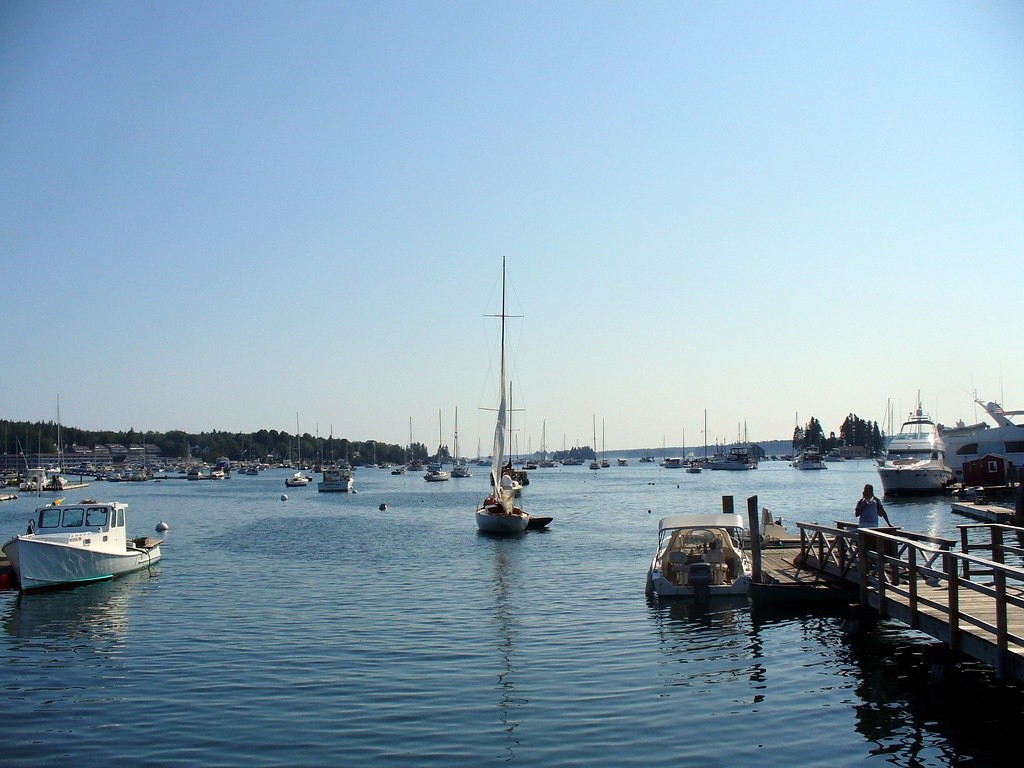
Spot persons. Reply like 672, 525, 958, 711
1006, 461, 1017, 486
703, 542, 726, 564
855, 484, 893, 569
26, 520, 35, 535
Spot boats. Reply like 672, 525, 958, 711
685, 460, 703, 472
2, 498, 163, 591
794, 444, 829, 471
317, 465, 354, 492
639, 455, 656, 463
618, 459, 627, 466
642, 513, 754, 600
526, 517, 554, 528
0, 407, 583, 489
658, 409, 760, 470
823, 447, 846, 462
876, 387, 953, 496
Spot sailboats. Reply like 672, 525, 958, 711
600, 417, 611, 467
472, 255, 531, 534
450, 405, 472, 478
285, 411, 309, 487
513, 418, 586, 470
588, 413, 601, 469
421, 408, 450, 481
